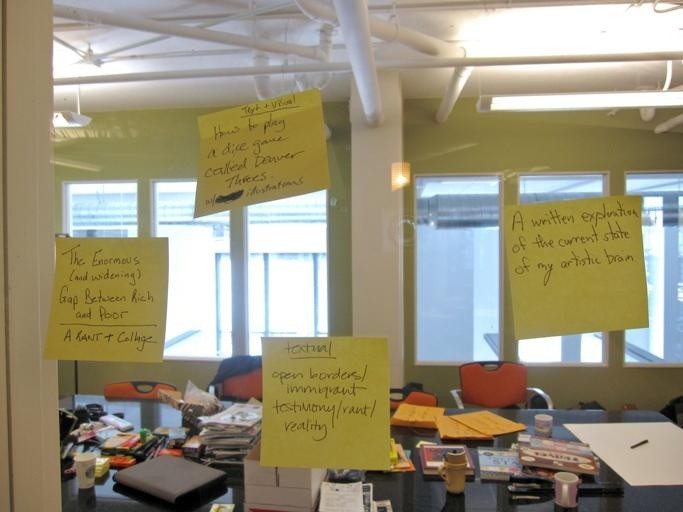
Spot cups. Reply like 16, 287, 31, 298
440, 454, 468, 494
534, 414, 554, 437
555, 471, 580, 508
73, 454, 96, 489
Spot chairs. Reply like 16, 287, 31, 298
451, 362, 554, 411
103, 381, 176, 399
209, 356, 263, 401
386, 389, 439, 408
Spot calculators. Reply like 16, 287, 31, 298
506, 475, 553, 504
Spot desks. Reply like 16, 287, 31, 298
58, 394, 683, 512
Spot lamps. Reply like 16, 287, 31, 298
476, 87, 683, 112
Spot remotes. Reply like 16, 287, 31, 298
99, 414, 133, 431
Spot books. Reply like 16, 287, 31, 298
113, 454, 228, 505
243, 442, 330, 512
197, 403, 263, 464
478, 432, 597, 485
419, 443, 475, 476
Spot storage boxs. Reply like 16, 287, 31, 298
243, 442, 330, 512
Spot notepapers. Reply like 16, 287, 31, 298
390, 403, 525, 441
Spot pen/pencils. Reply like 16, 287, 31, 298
630, 440, 648, 448
135, 436, 170, 463
579, 488, 624, 496
